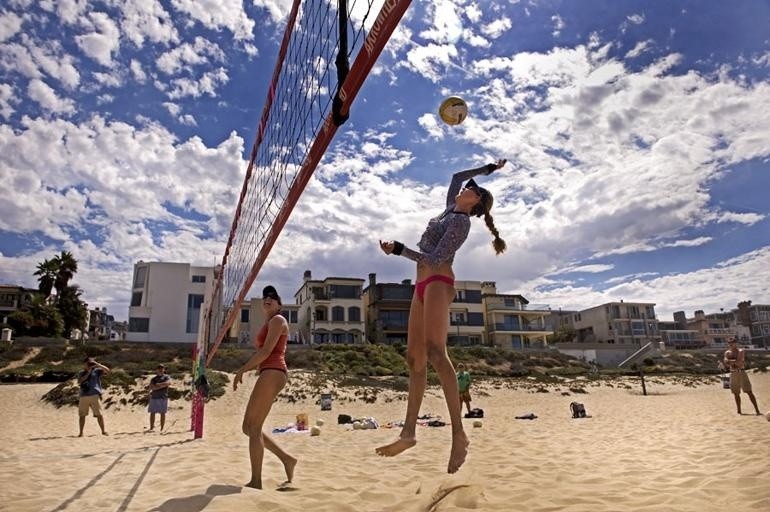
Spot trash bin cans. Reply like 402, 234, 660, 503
2, 328, 12, 341
322, 394, 331, 411
723, 377, 730, 389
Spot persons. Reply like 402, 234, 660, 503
76, 356, 111, 436
231, 285, 299, 491
722, 337, 761, 416
147, 362, 173, 432
374, 156, 509, 476
455, 362, 472, 417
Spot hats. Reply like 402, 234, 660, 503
262, 285, 281, 304
464, 178, 493, 218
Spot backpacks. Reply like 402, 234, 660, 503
465, 408, 484, 418
569, 402, 586, 419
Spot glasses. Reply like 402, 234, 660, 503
263, 293, 277, 300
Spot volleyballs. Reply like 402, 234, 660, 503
472, 419, 483, 428
311, 425, 322, 436
439, 95, 467, 125
316, 419, 324, 426
353, 422, 362, 430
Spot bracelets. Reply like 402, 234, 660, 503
485, 163, 497, 176
391, 240, 406, 256
